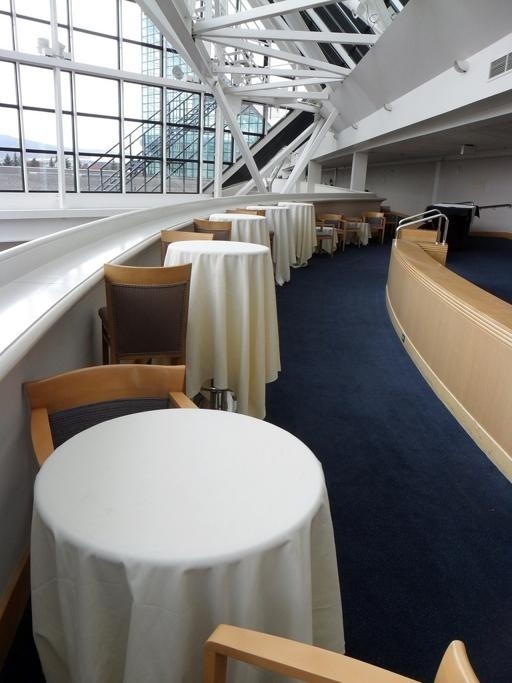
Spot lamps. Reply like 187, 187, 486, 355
384, 104, 392, 111
352, 121, 359, 129
454, 60, 469, 73
